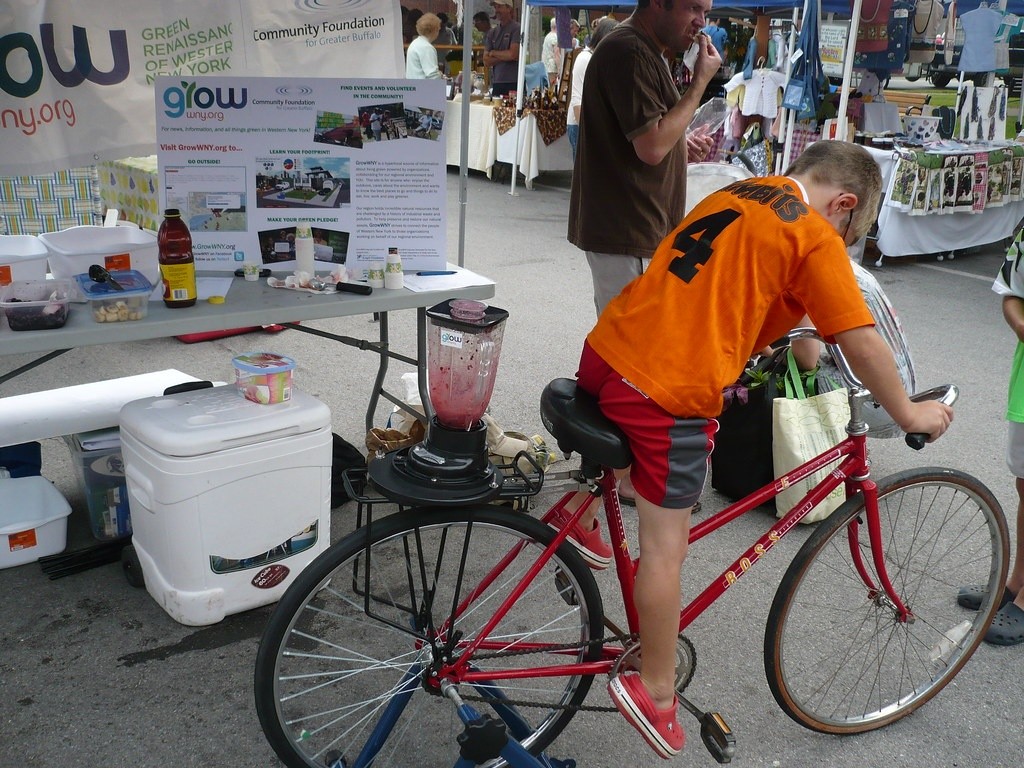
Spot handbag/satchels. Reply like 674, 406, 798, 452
782, 71, 831, 121
711, 346, 852, 524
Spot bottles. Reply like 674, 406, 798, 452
854, 132, 893, 149
455, 71, 464, 97
440, 74, 454, 100
504, 93, 517, 106
473, 74, 484, 93
551, 85, 560, 110
157, 209, 198, 307
534, 91, 542, 109
543, 91, 550, 110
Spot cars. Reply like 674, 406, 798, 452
922, 28, 1024, 98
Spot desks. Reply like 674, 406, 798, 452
441, 97, 575, 195
806, 135, 1024, 266
0, 264, 496, 454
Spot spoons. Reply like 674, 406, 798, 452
91, 265, 128, 290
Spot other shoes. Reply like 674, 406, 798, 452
617, 487, 702, 514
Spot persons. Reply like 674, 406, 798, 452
758, 258, 914, 438
700, 17, 729, 100
567, 0, 721, 324
401, 6, 458, 74
547, 140, 953, 759
957, 226, 1024, 646
413, 110, 432, 138
936, 35, 942, 42
567, 19, 621, 161
541, 17, 580, 87
482, 0, 520, 97
362, 107, 390, 141
405, 14, 443, 79
473, 12, 491, 66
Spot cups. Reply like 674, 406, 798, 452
482, 99, 502, 107
241, 261, 260, 281
368, 254, 404, 290
294, 222, 316, 278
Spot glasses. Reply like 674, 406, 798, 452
473, 21, 487, 24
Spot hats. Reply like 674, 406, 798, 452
489, 0, 514, 9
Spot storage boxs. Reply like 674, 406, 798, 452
118, 383, 333, 624
1, 225, 158, 322
0, 475, 72, 570
61, 433, 134, 541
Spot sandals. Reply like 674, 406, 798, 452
981, 600, 1024, 646
547, 507, 614, 570
956, 584, 1016, 612
606, 673, 686, 759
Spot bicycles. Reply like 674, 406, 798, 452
252, 320, 1014, 768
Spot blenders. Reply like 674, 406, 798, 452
365, 297, 504, 511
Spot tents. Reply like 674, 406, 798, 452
510, 0, 853, 193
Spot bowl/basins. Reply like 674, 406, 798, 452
900, 115, 943, 142
75, 269, 155, 322
0, 278, 74, 331
